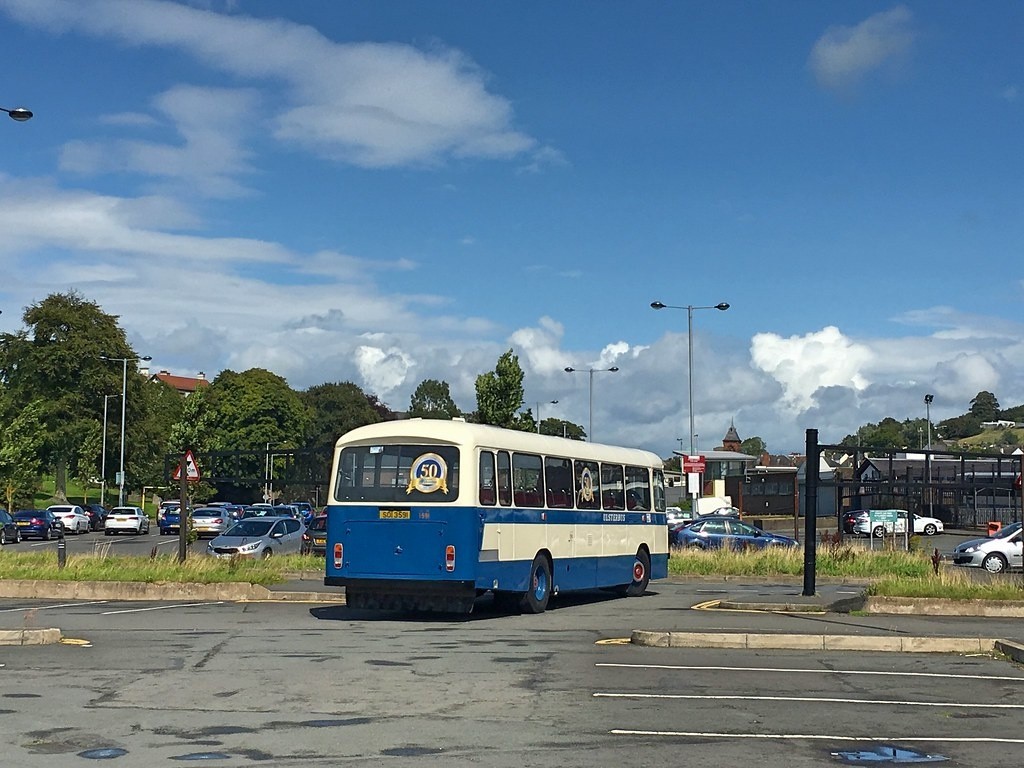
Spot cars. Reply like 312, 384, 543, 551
666, 507, 801, 551
842, 509, 945, 537
104, 506, 151, 536
0, 502, 103, 546
950, 521, 1024, 573
157, 500, 330, 565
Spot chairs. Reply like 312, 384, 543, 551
481, 485, 638, 510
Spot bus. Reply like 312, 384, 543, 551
324, 418, 671, 614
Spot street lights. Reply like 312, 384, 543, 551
99, 354, 152, 507
650, 300, 731, 456
564, 366, 619, 442
923, 394, 935, 517
676, 438, 684, 458
693, 434, 699, 451
264, 440, 296, 503
521, 401, 558, 434
